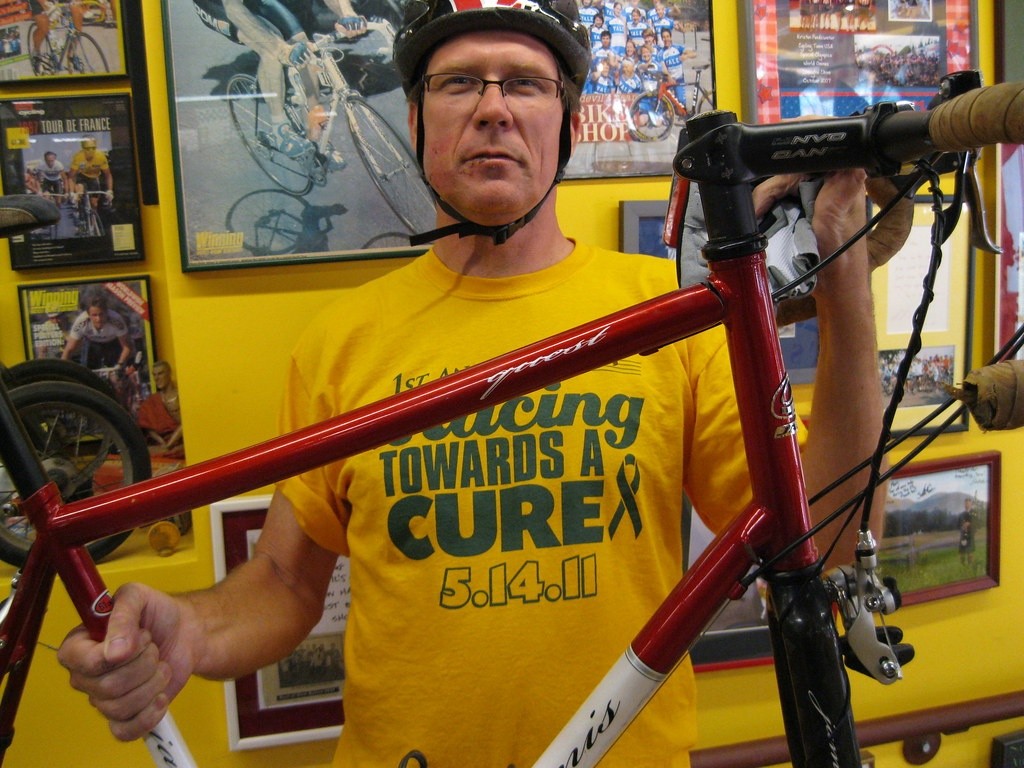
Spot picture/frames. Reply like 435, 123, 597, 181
881, 450, 1000, 608
618, 202, 872, 387
682, 501, 777, 672
210, 496, 352, 749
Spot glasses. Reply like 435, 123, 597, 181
83, 146, 97, 151
421, 72, 565, 114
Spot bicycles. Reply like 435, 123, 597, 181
71, 191, 113, 237
627, 64, 712, 143
0, 70, 1024, 768
227, 29, 438, 235
27, 2, 110, 77
38, 194, 69, 239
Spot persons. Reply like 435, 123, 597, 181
29, 0, 84, 76
800, 0, 938, 87
25, 136, 114, 237
878, 351, 951, 395
35, 294, 134, 410
56, 0, 891, 768
578, 0, 686, 125
192, 0, 367, 171
142, 360, 183, 450
956, 498, 980, 565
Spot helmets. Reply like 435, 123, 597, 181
391, 0, 592, 97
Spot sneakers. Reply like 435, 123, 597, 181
268, 119, 316, 159
318, 143, 346, 171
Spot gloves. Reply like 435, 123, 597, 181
70, 192, 78, 205
37, 191, 43, 197
105, 189, 114, 201
64, 192, 69, 198
334, 14, 368, 39
280, 40, 315, 72
